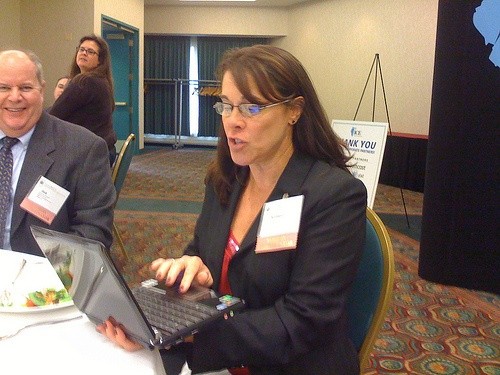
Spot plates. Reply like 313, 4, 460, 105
0, 269, 75, 313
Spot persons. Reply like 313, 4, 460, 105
96, 46, 368, 375
54, 77, 71, 99
52, 36, 117, 167
0, 50, 116, 258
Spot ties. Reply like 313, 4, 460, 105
0, 136, 20, 250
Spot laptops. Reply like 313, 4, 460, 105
29, 224, 247, 351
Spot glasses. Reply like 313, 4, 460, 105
76, 47, 99, 57
212, 98, 294, 119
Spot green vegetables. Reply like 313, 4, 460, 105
28, 289, 71, 307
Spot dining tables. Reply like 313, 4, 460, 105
0, 248, 167, 375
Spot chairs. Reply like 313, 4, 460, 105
110, 133, 136, 264
359, 206, 395, 375
